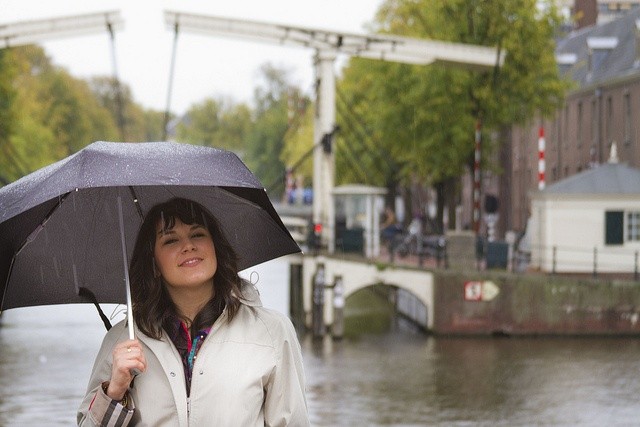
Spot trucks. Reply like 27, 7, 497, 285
281, 217, 307, 241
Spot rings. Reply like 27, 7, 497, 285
126, 345, 132, 352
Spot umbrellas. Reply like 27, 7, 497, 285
0, 138, 305, 375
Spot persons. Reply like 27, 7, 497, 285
77, 197, 313, 427
379, 205, 398, 264
